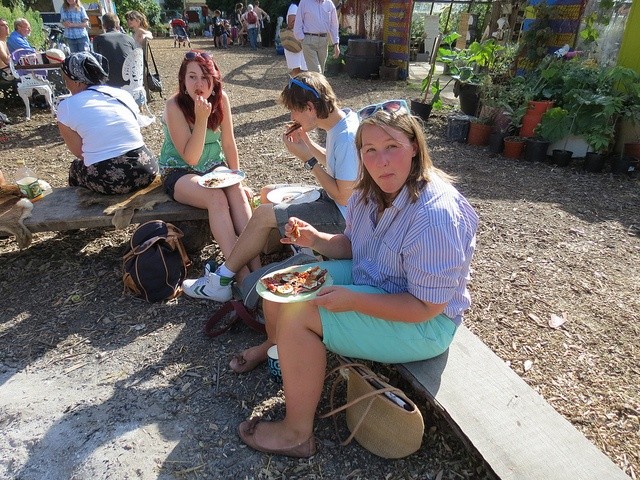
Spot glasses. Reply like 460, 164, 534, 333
357, 99, 409, 123
287, 73, 319, 98
126, 16, 136, 21
184, 52, 213, 62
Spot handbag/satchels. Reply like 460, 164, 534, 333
279, 26, 302, 53
145, 41, 163, 100
316, 355, 426, 458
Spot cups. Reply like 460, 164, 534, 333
25, 55, 37, 66
18, 57, 27, 66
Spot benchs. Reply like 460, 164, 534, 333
3, 188, 232, 255
269, 185, 633, 477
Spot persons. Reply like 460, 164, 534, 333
57, 52, 157, 197
234, 2, 246, 44
0, 23, 11, 71
121, 9, 151, 48
92, 9, 138, 87
281, 0, 303, 78
227, 102, 478, 460
160, 49, 262, 284
241, 3, 259, 49
57, 0, 92, 56
238, 21, 247, 35
293, 1, 342, 78
7, 18, 54, 82
251, 0, 268, 49
212, 9, 226, 49
182, 73, 366, 304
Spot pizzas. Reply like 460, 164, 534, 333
285, 219, 303, 242
285, 122, 302, 136
259, 264, 328, 298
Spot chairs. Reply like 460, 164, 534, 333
106, 48, 156, 110
47, 23, 65, 45
143, 37, 153, 104
9, 49, 57, 120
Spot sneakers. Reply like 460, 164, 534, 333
237, 416, 316, 458
181, 263, 232, 302
229, 345, 268, 373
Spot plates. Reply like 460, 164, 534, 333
255, 267, 334, 304
266, 187, 321, 206
198, 169, 246, 189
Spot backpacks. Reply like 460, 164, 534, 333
120, 218, 192, 302
204, 251, 319, 338
246, 11, 258, 24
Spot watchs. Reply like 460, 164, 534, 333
304, 157, 318, 171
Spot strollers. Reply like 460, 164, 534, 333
171, 19, 190, 48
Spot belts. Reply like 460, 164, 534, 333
305, 32, 328, 37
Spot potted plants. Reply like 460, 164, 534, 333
326, 45, 345, 74
410, 31, 497, 116
535, 84, 595, 167
585, 66, 626, 170
470, 79, 517, 145
501, 94, 531, 158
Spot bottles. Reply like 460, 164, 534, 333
15, 160, 43, 202
